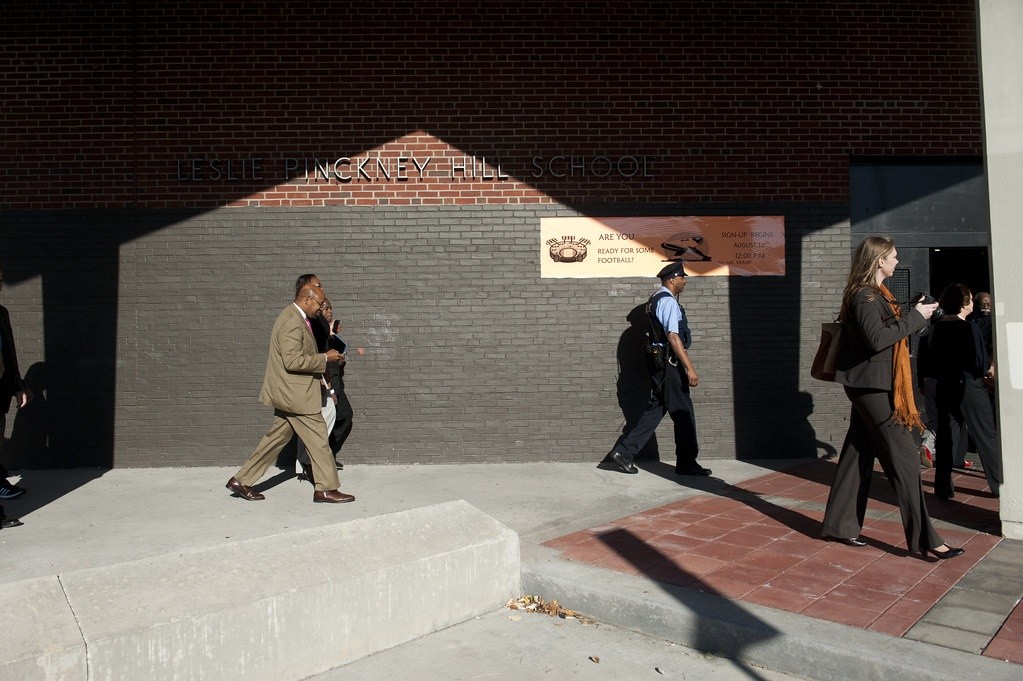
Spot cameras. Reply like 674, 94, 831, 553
912, 293, 945, 324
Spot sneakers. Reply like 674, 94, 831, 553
0, 478, 26, 499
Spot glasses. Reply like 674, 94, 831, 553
313, 298, 323, 307
315, 283, 322, 289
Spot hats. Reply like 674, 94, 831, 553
657, 261, 688, 282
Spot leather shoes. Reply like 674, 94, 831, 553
0, 517, 21, 528
676, 462, 712, 476
609, 450, 638, 474
226, 476, 265, 501
313, 489, 355, 503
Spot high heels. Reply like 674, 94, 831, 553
827, 536, 867, 546
922, 544, 964, 559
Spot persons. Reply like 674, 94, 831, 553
810, 235, 965, 561
916, 284, 1003, 510
0, 281, 29, 528
225, 273, 355, 504
596, 262, 712, 477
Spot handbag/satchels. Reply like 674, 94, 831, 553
811, 312, 843, 381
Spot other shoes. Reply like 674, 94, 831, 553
920, 446, 933, 468
302, 463, 314, 484
964, 460, 973, 468
335, 461, 343, 468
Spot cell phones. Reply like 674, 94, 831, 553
342, 352, 347, 355
